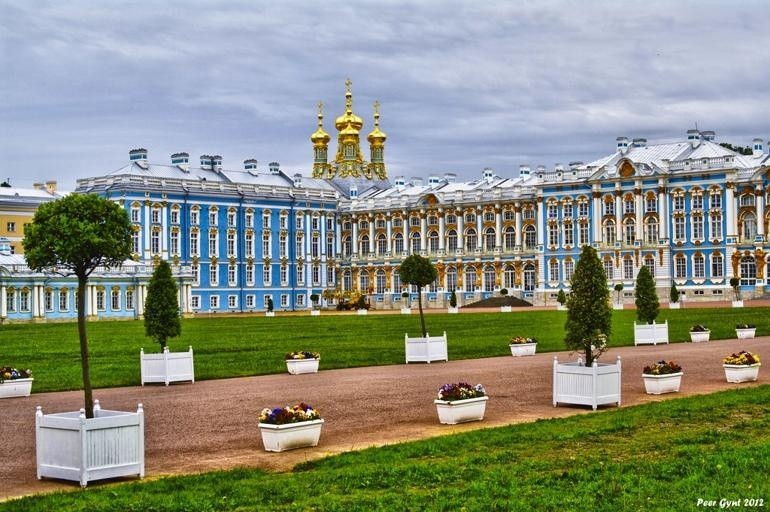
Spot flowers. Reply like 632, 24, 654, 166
436, 381, 486, 402
641, 360, 682, 376
736, 324, 755, 329
689, 325, 709, 332
258, 402, 321, 426
285, 350, 320, 360
721, 349, 760, 368
0, 366, 33, 384
510, 336, 537, 345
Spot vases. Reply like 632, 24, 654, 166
258, 418, 325, 452
0, 378, 34, 398
508, 343, 537, 357
283, 358, 319, 375
434, 396, 489, 425
688, 330, 711, 342
722, 362, 761, 383
642, 372, 683, 395
735, 328, 756, 339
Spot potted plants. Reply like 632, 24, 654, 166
499, 288, 512, 313
632, 264, 680, 347
397, 253, 457, 364
265, 298, 276, 317
551, 245, 624, 412
357, 295, 368, 314
139, 260, 195, 388
309, 294, 320, 315
730, 277, 744, 308
20, 191, 146, 492
